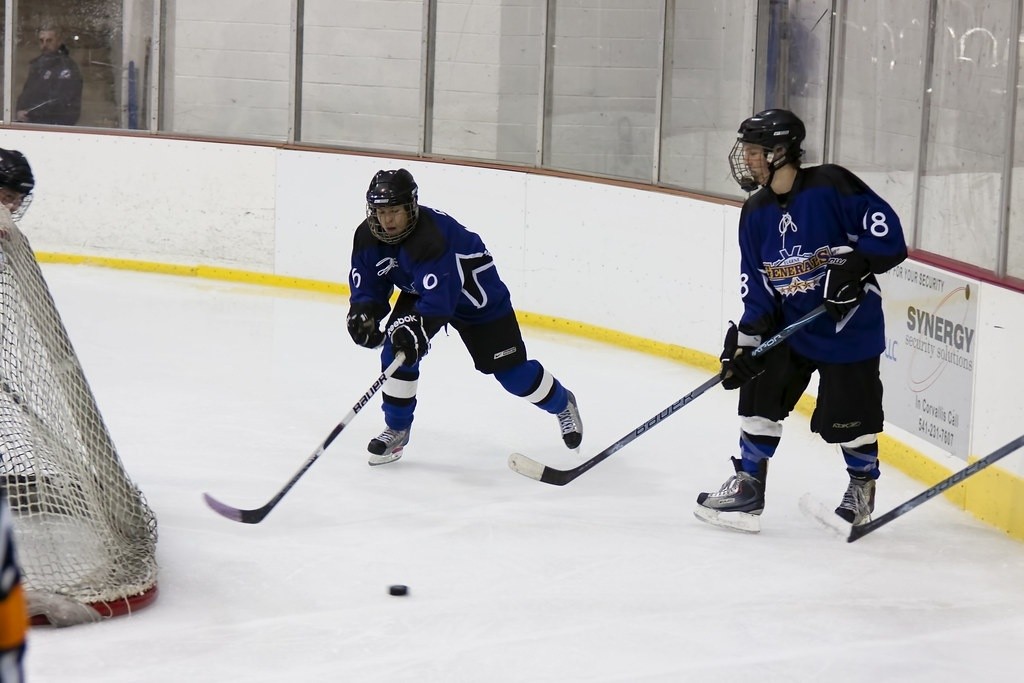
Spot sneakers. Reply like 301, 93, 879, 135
694, 457, 768, 535
834, 469, 876, 528
557, 390, 583, 453
367, 426, 411, 467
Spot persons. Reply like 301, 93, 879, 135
0, 148, 37, 221
695, 108, 909, 524
16, 21, 83, 127
346, 168, 584, 454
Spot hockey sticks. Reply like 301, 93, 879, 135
506, 305, 827, 486
202, 350, 406, 525
798, 432, 1022, 543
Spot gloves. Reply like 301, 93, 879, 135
392, 328, 430, 365
825, 255, 866, 322
346, 312, 386, 349
721, 321, 766, 390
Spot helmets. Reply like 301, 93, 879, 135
366, 169, 418, 242
0, 147, 34, 222
728, 109, 805, 192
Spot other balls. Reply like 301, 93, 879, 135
388, 584, 410, 598
